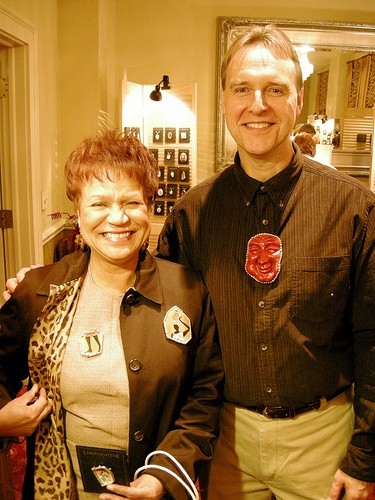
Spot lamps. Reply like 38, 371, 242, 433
149, 73, 171, 102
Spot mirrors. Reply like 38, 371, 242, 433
212, 13, 375, 183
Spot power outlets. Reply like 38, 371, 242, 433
40, 190, 49, 212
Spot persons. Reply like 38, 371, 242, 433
295, 124, 339, 170
0, 132, 224, 500
3, 26, 375, 500
